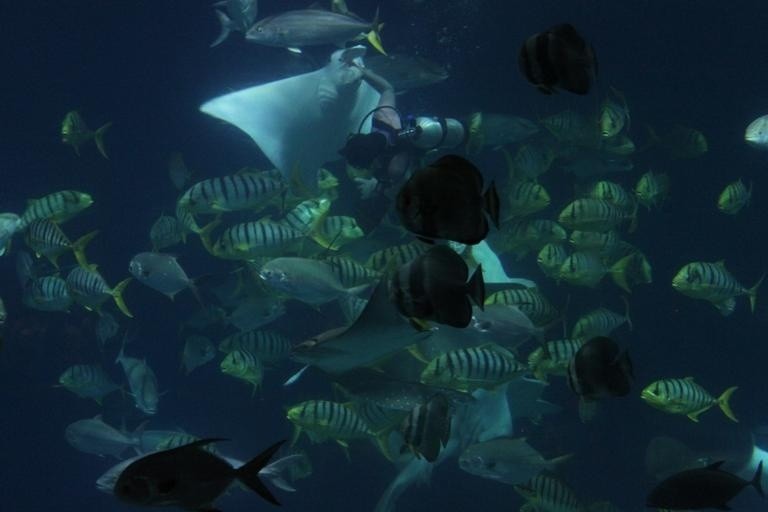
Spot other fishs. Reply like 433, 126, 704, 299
0, 98, 768, 511
